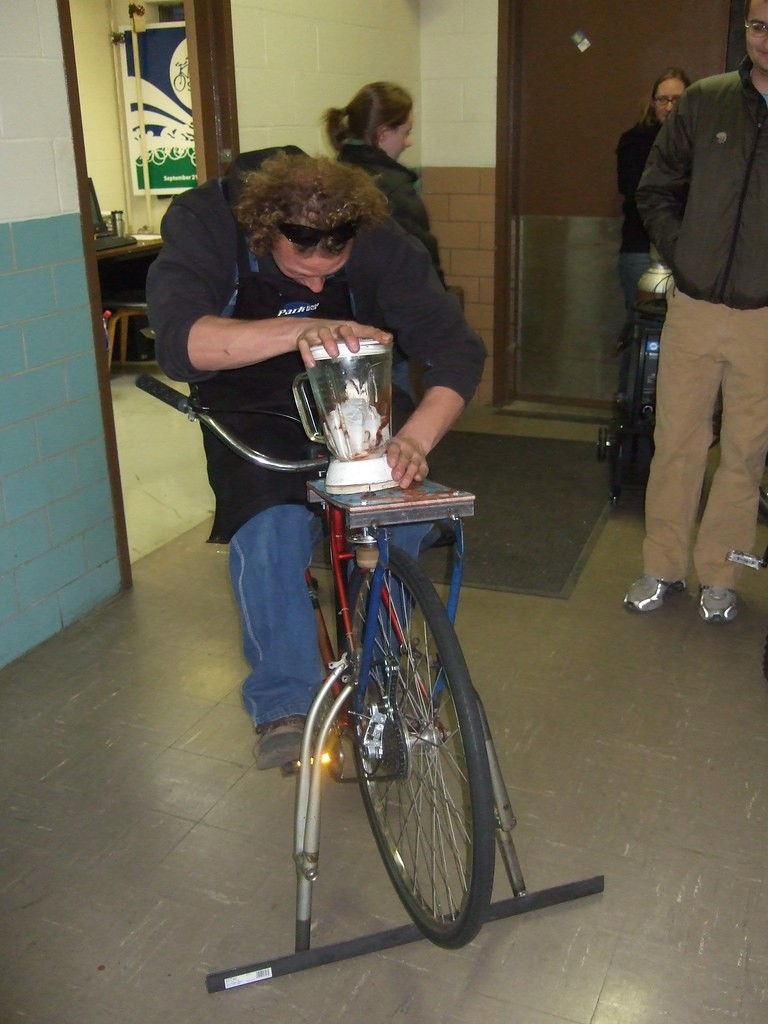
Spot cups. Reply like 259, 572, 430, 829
111, 210, 124, 237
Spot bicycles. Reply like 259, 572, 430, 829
133, 368, 606, 994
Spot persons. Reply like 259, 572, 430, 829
614, 0, 768, 623
147, 82, 490, 768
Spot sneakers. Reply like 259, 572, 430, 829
623, 575, 686, 613
697, 582, 738, 623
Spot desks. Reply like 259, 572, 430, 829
94, 231, 165, 267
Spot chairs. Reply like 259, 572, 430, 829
105, 300, 157, 371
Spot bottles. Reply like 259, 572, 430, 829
103, 310, 111, 329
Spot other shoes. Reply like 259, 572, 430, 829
428, 529, 456, 547
253, 714, 319, 771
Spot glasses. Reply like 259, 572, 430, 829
745, 18, 768, 38
653, 94, 680, 107
276, 216, 362, 247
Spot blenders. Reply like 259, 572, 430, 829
293, 335, 399, 495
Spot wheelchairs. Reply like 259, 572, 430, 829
594, 303, 767, 527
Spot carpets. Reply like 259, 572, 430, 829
308, 432, 620, 601
